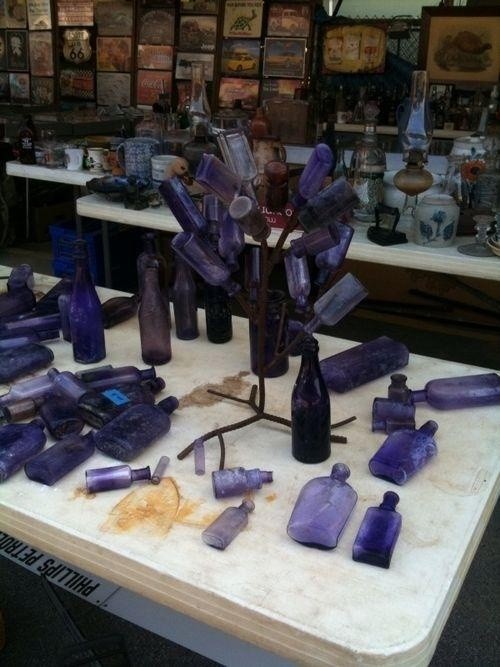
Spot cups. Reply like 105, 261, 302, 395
115, 136, 160, 179
87, 147, 110, 173
251, 134, 287, 175
64, 149, 84, 171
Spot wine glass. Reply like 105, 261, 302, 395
41, 128, 57, 165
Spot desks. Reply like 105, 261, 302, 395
77, 192, 500, 290
1, 266, 500, 666
6, 159, 113, 240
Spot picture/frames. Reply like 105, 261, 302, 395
1, 0, 313, 120
416, 6, 498, 87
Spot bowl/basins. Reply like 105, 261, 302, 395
85, 174, 151, 203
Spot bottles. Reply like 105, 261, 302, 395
262, 160, 289, 213
369, 420, 440, 485
2, 236, 200, 487
286, 462, 359, 551
195, 152, 247, 206
362, 373, 414, 434
410, 372, 498, 411
201, 495, 262, 551
346, 117, 386, 223
194, 437, 207, 475
250, 106, 273, 138
84, 464, 151, 496
186, 60, 212, 128
354, 489, 403, 567
291, 338, 332, 463
215, 128, 259, 206
134, 114, 162, 142
164, 178, 371, 337
293, 143, 335, 206
212, 466, 275, 499
319, 336, 409, 394
398, 70, 435, 164
16, 117, 37, 164
